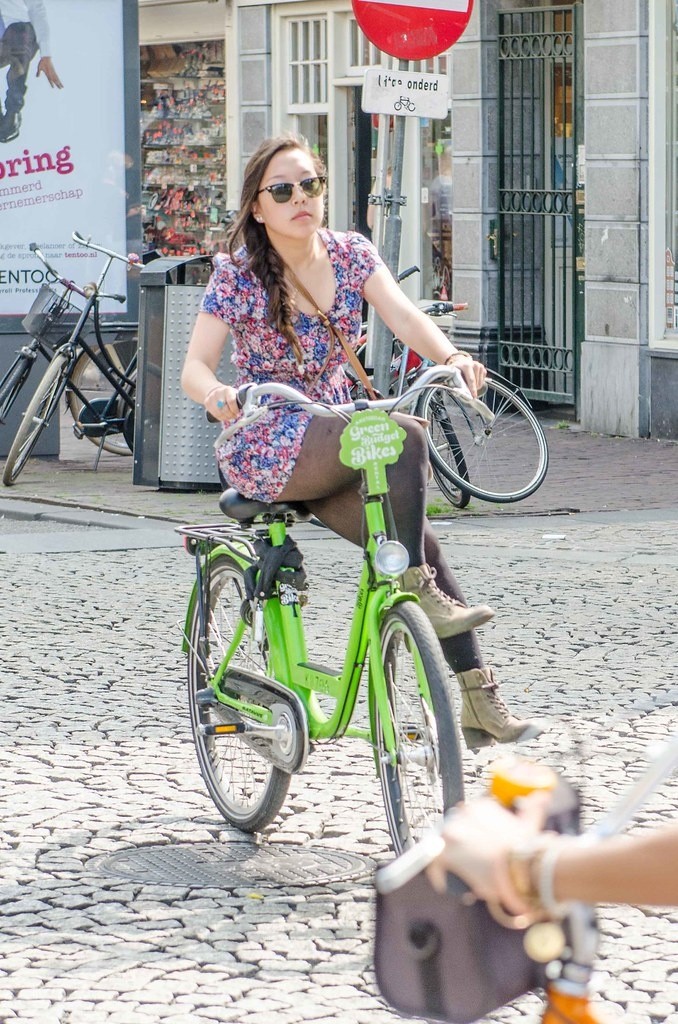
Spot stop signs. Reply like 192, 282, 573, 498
349, 0, 475, 61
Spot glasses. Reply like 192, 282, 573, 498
259, 176, 328, 203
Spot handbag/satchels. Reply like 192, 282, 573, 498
372, 765, 594, 1024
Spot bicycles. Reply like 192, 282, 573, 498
176, 360, 497, 859
2, 228, 548, 511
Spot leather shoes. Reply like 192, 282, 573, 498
0, 111, 23, 144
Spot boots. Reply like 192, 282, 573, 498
453, 667, 544, 750
397, 562, 497, 643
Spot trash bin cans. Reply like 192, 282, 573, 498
130, 248, 244, 492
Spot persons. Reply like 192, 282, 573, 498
182, 139, 537, 749
366, 164, 392, 243
0, 0, 64, 143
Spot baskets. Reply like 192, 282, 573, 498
21, 285, 96, 355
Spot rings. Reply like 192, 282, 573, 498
217, 400, 226, 409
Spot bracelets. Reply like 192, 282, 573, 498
207, 386, 221, 396
445, 352, 470, 365
